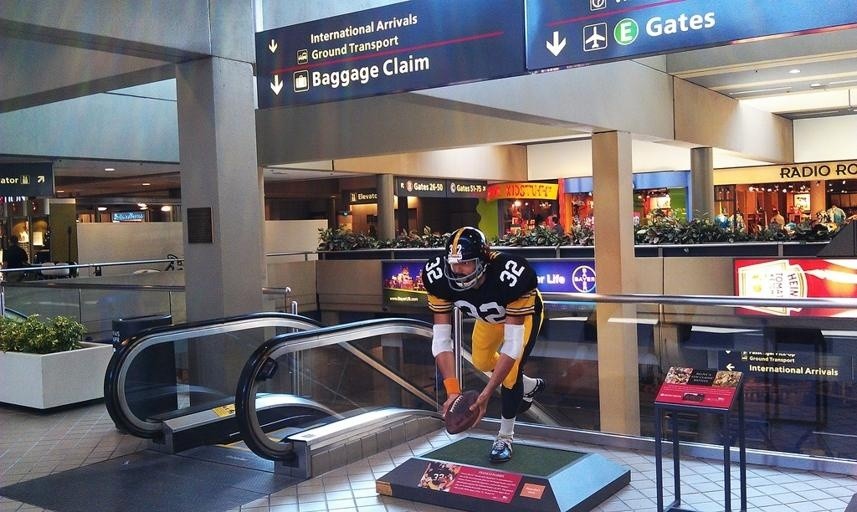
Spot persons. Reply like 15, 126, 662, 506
3, 233, 29, 282
548, 216, 564, 237
418, 224, 547, 465
826, 204, 845, 224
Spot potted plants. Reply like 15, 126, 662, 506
0, 312, 113, 410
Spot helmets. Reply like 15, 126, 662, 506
443, 226, 490, 292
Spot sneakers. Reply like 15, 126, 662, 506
516, 377, 545, 414
488, 431, 515, 462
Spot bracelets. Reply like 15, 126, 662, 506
440, 376, 463, 396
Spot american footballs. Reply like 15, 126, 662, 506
445, 390, 480, 434
